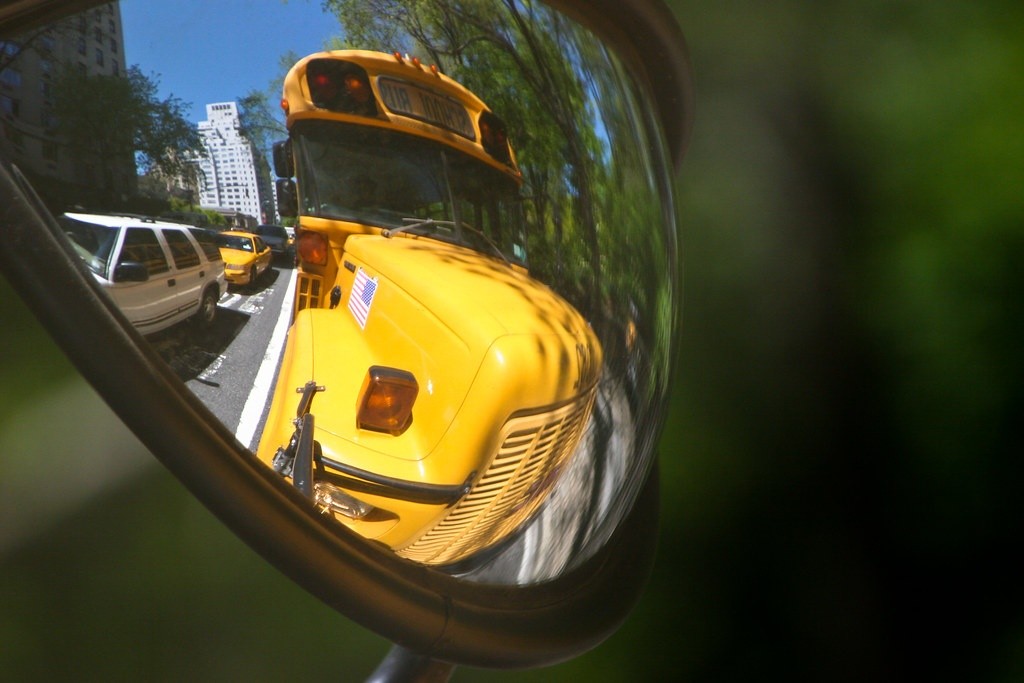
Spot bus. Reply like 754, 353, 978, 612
252, 50, 604, 567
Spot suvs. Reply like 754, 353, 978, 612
61, 210, 228, 336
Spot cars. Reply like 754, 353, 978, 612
216, 231, 272, 289
254, 225, 289, 258
